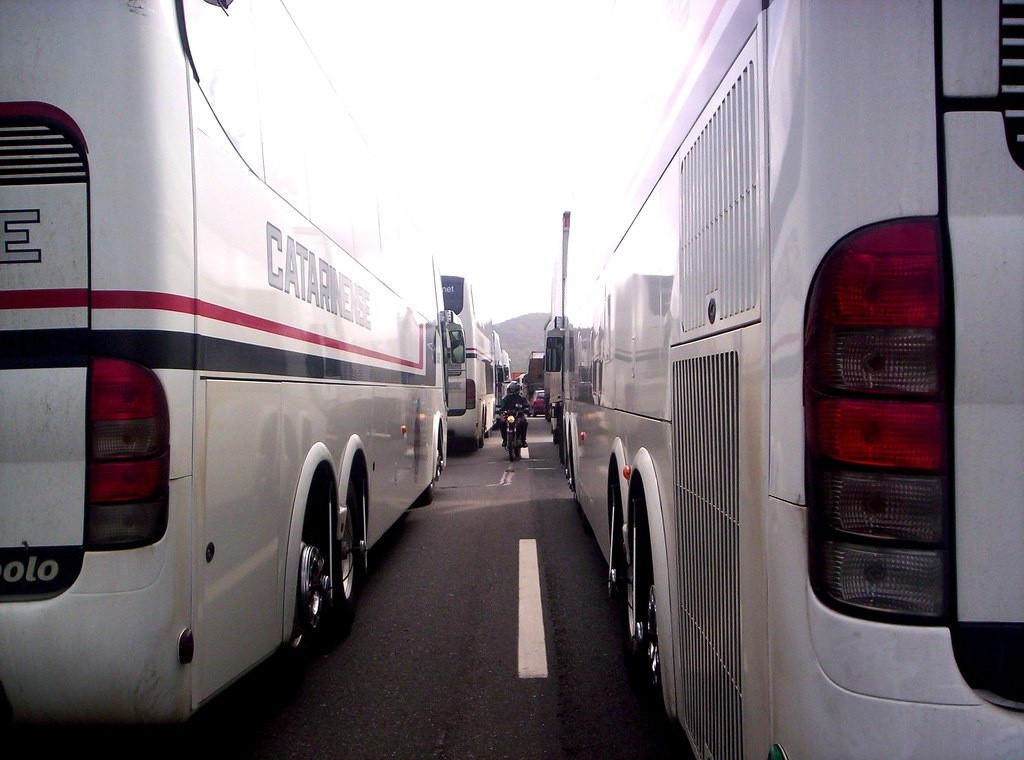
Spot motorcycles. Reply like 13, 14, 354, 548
494, 404, 531, 463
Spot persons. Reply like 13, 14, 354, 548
495, 381, 530, 448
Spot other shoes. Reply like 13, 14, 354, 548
522, 440, 528, 448
502, 440, 507, 447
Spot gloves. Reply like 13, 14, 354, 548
524, 410, 528, 413
500, 409, 508, 414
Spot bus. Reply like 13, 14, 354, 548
514, 314, 562, 444
445, 278, 511, 452
546, 0, 1024, 760
1, 3, 467, 728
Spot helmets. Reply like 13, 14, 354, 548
507, 381, 520, 396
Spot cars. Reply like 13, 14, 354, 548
529, 388, 548, 418
